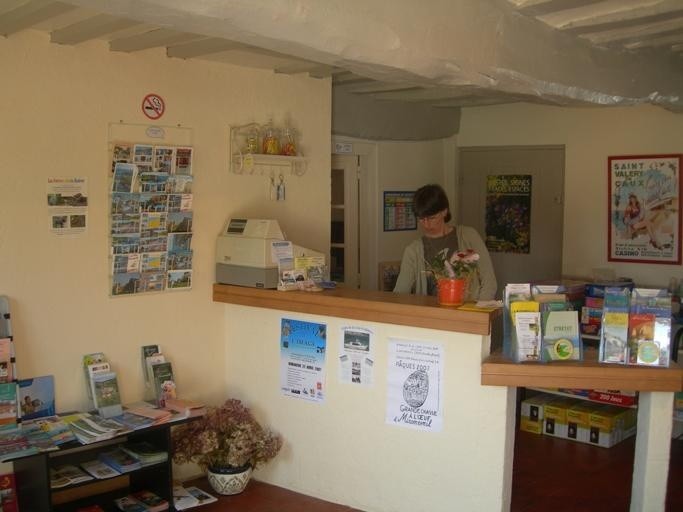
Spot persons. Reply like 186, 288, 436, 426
623, 194, 658, 250
394, 182, 497, 301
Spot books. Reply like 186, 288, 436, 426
503, 280, 672, 370
1, 344, 219, 512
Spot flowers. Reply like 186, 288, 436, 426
418, 247, 480, 280
173, 397, 284, 472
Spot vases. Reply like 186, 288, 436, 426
437, 277, 466, 306
207, 465, 254, 496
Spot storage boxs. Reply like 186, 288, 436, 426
520, 394, 637, 449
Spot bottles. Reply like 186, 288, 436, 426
245, 126, 297, 157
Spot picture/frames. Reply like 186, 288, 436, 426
607, 154, 682, 266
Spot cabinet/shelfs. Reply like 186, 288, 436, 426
0, 398, 211, 512
